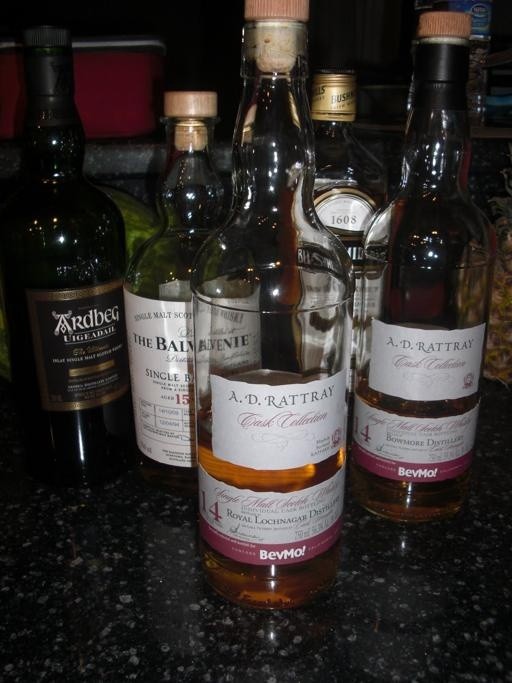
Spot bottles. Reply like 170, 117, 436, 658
307, 70, 388, 406
349, 13, 490, 524
14, 25, 136, 489
146, 89, 222, 241
1, 141, 41, 428
188, 2, 358, 604
118, 92, 265, 528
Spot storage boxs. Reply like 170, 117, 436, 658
0, 35, 167, 140
